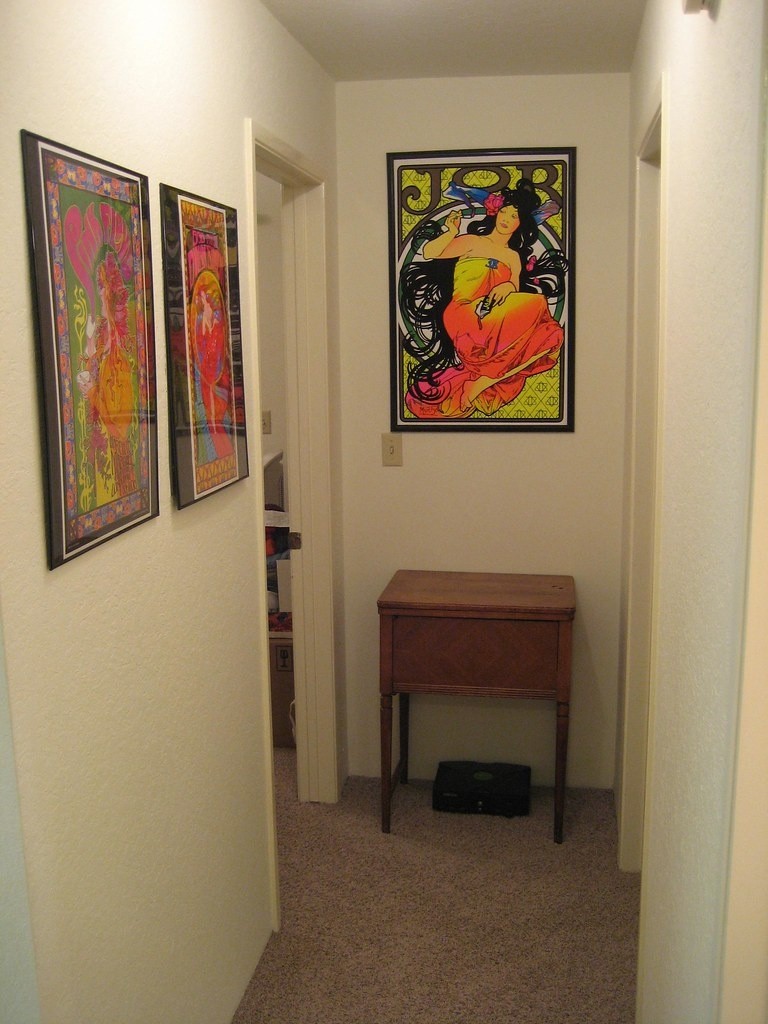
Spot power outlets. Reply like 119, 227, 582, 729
263, 410, 270, 433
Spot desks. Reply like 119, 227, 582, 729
377, 569, 576, 844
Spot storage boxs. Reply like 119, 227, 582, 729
270, 639, 296, 748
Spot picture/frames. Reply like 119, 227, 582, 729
20, 129, 159, 570
158, 182, 249, 510
386, 147, 576, 432
